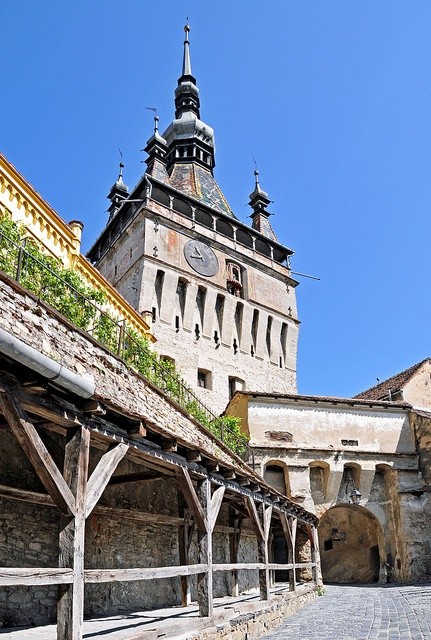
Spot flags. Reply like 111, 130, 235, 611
118, 147, 122, 157
140, 161, 146, 163
140, 148, 145, 151
252, 155, 257, 167
144, 107, 156, 111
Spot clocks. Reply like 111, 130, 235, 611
184, 240, 219, 277
